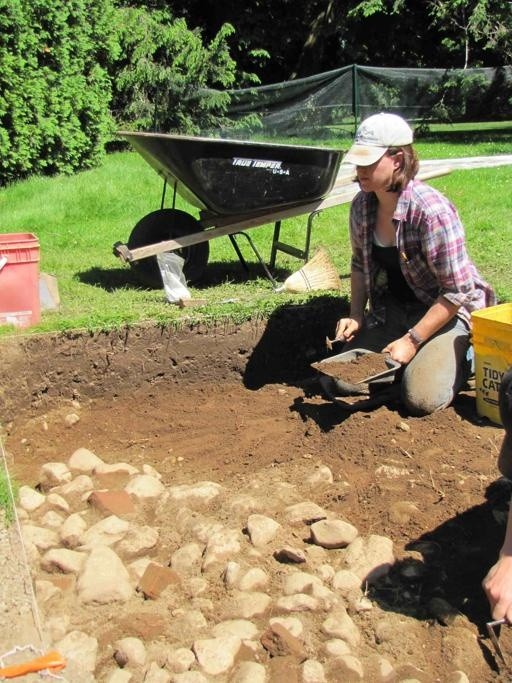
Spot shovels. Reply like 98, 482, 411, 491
485, 619, 508, 667
310, 348, 402, 386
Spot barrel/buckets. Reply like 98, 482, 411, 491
468, 303, 512, 423
0, 232, 41, 325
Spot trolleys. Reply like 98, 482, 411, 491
111, 127, 454, 290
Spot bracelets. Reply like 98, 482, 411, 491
407, 328, 425, 345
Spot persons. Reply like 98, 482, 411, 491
481, 367, 512, 625
334, 112, 497, 415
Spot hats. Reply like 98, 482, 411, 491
340, 111, 415, 166
